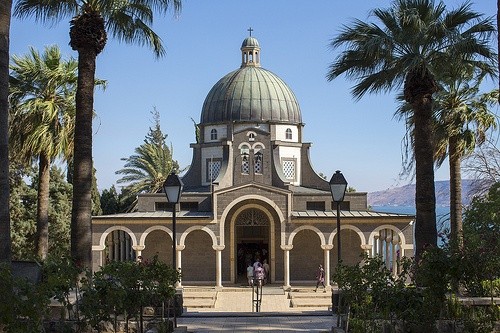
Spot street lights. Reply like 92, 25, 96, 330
163, 171, 184, 329
328, 169, 348, 329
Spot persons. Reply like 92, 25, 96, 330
238, 247, 267, 269
246, 263, 255, 286
313, 264, 328, 293
262, 259, 270, 284
255, 263, 264, 285
253, 259, 262, 270
